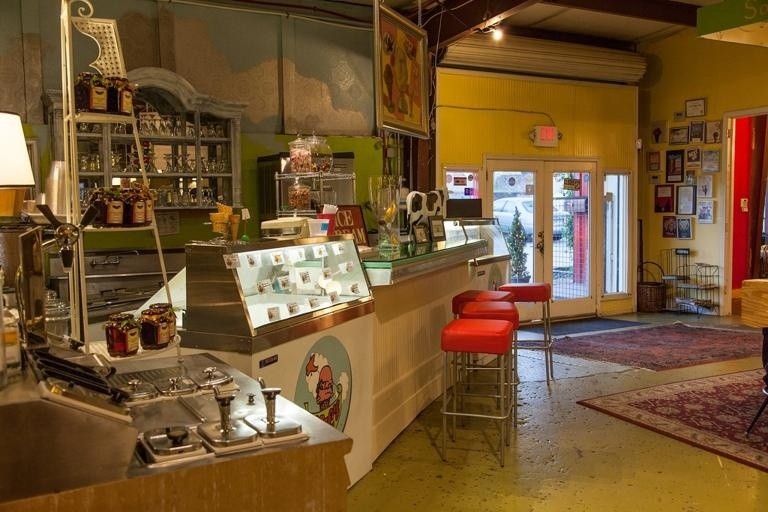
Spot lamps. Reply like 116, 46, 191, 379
0, 109, 37, 189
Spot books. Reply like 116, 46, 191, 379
676, 297, 712, 307
661, 274, 685, 280
679, 283, 714, 289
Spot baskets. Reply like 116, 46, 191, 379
637, 261, 667, 313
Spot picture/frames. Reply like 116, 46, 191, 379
644, 96, 726, 241
373, 1, 431, 141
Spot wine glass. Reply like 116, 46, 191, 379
78, 113, 232, 174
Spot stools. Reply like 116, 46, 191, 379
439, 317, 513, 469
497, 283, 559, 386
454, 301, 523, 429
450, 288, 516, 391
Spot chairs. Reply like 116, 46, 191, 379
745, 325, 767, 438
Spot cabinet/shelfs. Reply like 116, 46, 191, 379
673, 263, 721, 318
269, 168, 358, 220
178, 229, 377, 500
71, 126, 233, 214
657, 246, 691, 315
442, 216, 518, 370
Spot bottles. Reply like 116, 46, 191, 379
288, 131, 333, 175
286, 176, 312, 210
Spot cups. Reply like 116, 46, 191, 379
24, 159, 69, 215
375, 188, 400, 251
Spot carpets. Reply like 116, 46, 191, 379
519, 318, 765, 372
573, 365, 767, 476
517, 315, 652, 337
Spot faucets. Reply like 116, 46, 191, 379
104, 250, 140, 266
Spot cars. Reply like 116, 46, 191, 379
447, 173, 569, 240
